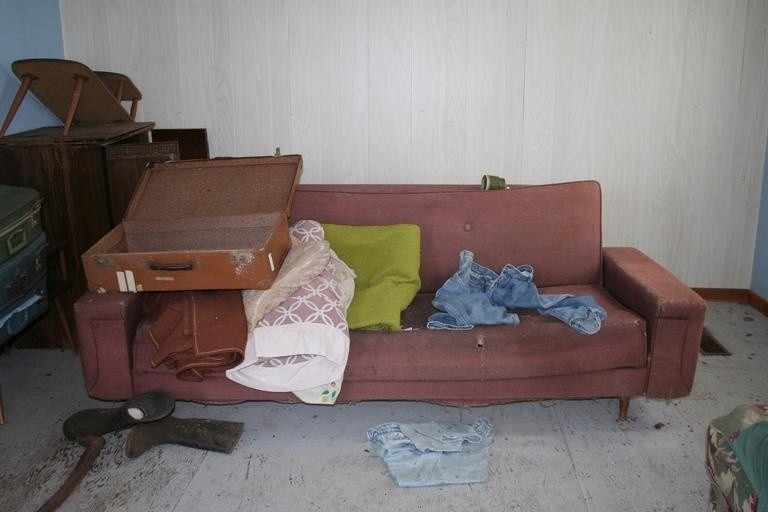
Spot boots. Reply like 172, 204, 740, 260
63, 390, 174, 441
125, 417, 244, 459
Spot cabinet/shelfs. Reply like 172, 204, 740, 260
3, 119, 211, 351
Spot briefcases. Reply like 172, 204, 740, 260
81, 154, 303, 293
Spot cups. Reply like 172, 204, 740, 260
480, 175, 506, 191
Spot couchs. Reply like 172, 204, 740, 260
70, 179, 702, 426
